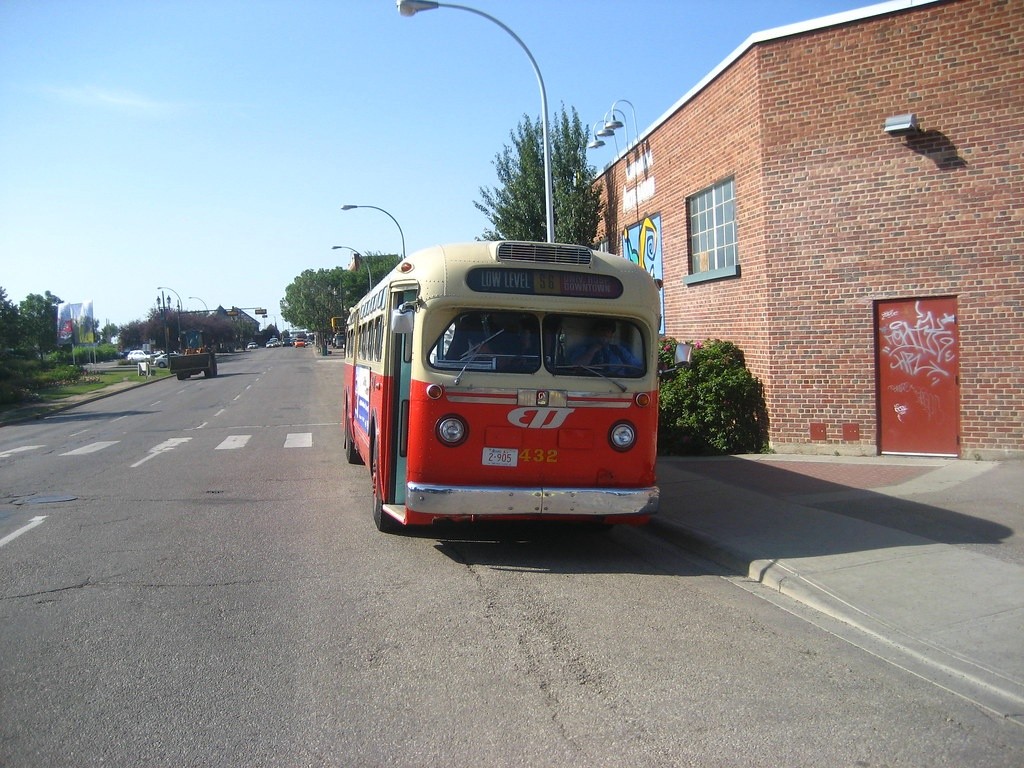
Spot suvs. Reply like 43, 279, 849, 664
127, 350, 160, 360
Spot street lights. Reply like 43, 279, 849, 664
341, 204, 405, 259
189, 297, 208, 310
395, 0, 554, 243
158, 286, 182, 311
331, 246, 371, 291
156, 291, 171, 369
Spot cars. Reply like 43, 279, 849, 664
247, 342, 258, 349
266, 337, 312, 348
153, 353, 183, 368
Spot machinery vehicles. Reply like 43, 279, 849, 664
168, 328, 217, 381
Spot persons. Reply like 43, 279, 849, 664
566, 319, 643, 376
471, 334, 506, 367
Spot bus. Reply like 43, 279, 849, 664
342, 240, 693, 533
